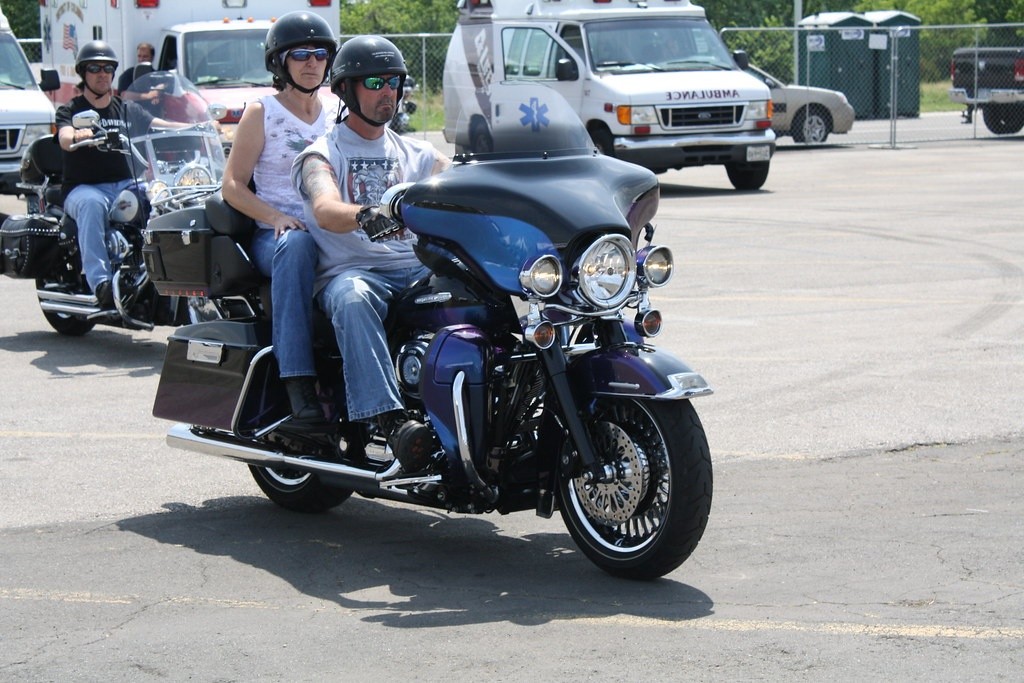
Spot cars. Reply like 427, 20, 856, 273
665, 58, 854, 143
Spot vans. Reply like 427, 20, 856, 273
440, 0, 778, 192
0, 8, 62, 194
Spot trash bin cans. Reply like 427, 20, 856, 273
797, 12, 874, 119
864, 10, 922, 120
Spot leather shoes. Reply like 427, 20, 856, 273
284, 378, 326, 424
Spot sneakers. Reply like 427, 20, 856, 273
376, 411, 432, 474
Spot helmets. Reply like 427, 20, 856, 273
329, 35, 408, 92
264, 11, 337, 70
75, 40, 118, 72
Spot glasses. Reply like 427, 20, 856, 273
286, 48, 328, 61
85, 64, 115, 73
356, 76, 400, 90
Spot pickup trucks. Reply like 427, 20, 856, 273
945, 42, 1024, 134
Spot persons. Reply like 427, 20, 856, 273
56, 40, 223, 312
222, 11, 347, 428
117, 42, 161, 119
661, 40, 679, 57
291, 36, 452, 474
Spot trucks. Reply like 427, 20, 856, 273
38, 0, 344, 205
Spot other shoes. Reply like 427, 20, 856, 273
96, 280, 114, 309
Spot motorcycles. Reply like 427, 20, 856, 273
139, 16, 718, 583
0, 69, 240, 337
